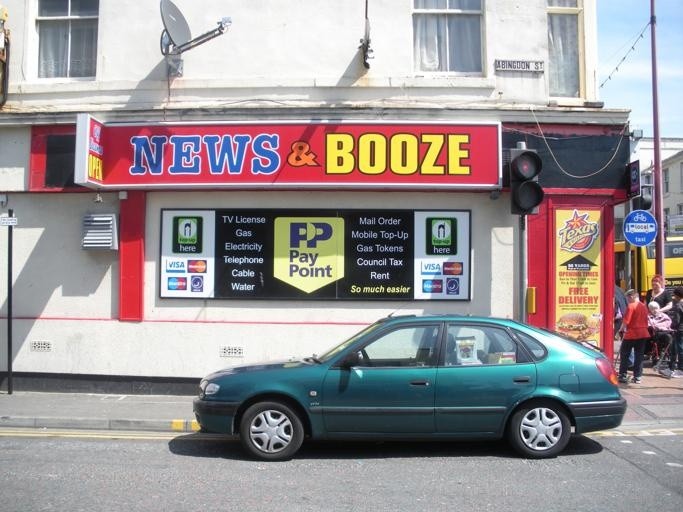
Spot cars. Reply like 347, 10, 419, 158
193, 315, 627, 460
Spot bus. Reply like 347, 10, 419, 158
613, 236, 683, 304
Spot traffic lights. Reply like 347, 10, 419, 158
508, 147, 545, 217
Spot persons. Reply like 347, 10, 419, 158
614, 274, 683, 384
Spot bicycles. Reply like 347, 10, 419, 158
644, 325, 678, 378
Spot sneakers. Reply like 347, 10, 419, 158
618, 373, 641, 384
